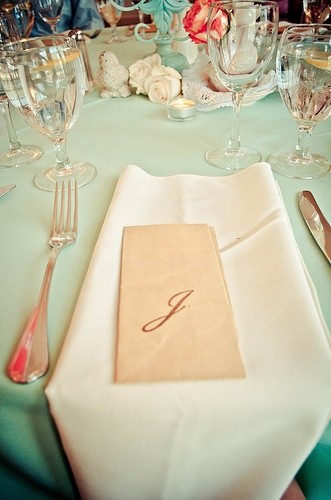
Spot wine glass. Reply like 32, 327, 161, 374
204, 0, 279, 171
95, 0, 128, 43
34, 0, 64, 35
0, 36, 95, 192
0, 43, 43, 167
302, 0, 331, 40
131, 0, 151, 39
267, 23, 331, 180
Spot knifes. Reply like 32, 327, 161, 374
299, 190, 331, 264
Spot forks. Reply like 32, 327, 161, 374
7, 182, 78, 383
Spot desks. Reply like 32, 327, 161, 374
0, 23, 331, 500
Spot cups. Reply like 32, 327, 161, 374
0, 0, 34, 39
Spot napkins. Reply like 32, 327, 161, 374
44, 161, 331, 500
114, 223, 243, 382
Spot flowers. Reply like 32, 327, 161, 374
129, 53, 184, 102
183, 0, 233, 47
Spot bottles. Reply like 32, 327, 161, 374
69, 29, 96, 93
